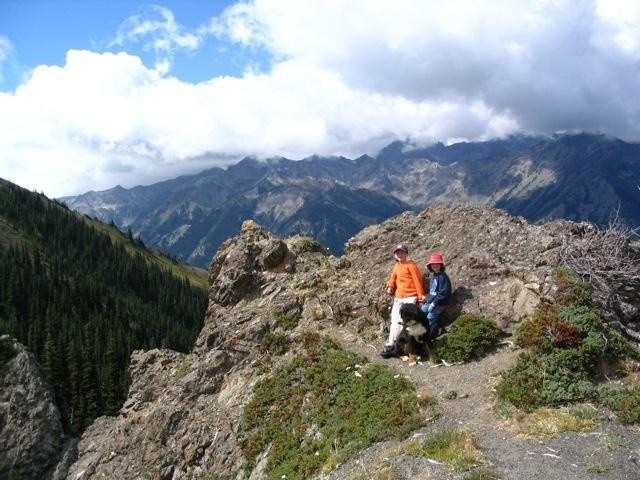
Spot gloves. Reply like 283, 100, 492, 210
428, 302, 435, 312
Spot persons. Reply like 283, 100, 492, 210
420, 249, 449, 334
380, 243, 423, 355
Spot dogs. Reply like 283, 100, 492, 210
379, 303, 439, 367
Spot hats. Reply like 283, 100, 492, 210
426, 253, 444, 266
394, 245, 408, 253
